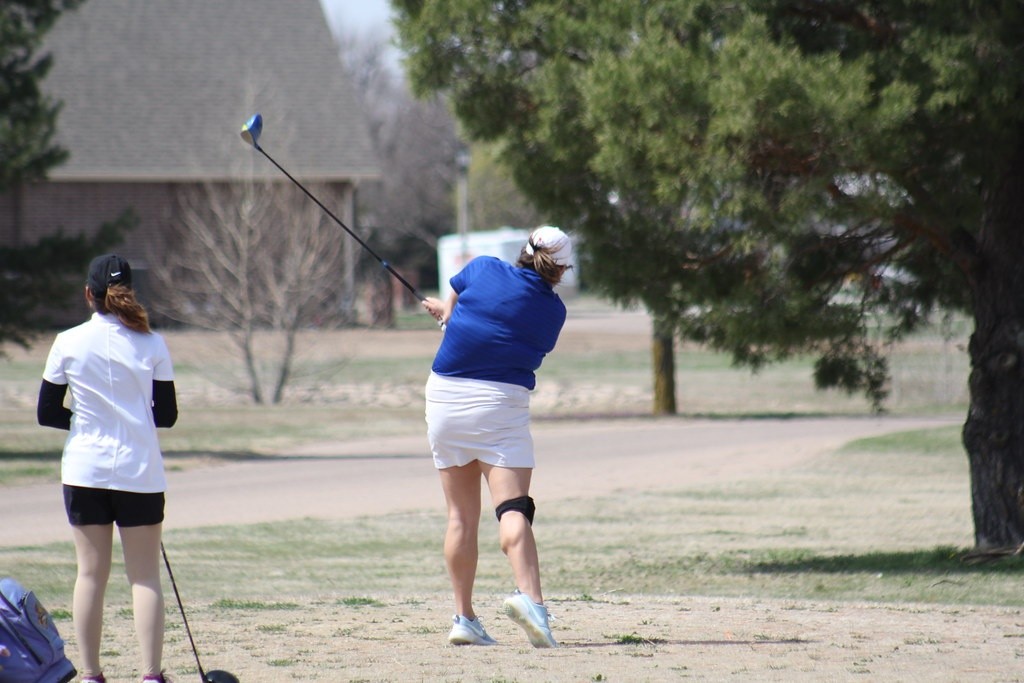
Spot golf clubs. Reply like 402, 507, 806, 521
240, 112, 427, 302
160, 541, 240, 683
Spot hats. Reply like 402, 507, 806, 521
527, 225, 570, 270
87, 255, 134, 295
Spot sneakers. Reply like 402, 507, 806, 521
450, 614, 498, 645
506, 588, 557, 648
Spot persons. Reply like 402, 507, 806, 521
36, 253, 178, 683
421, 227, 572, 649
